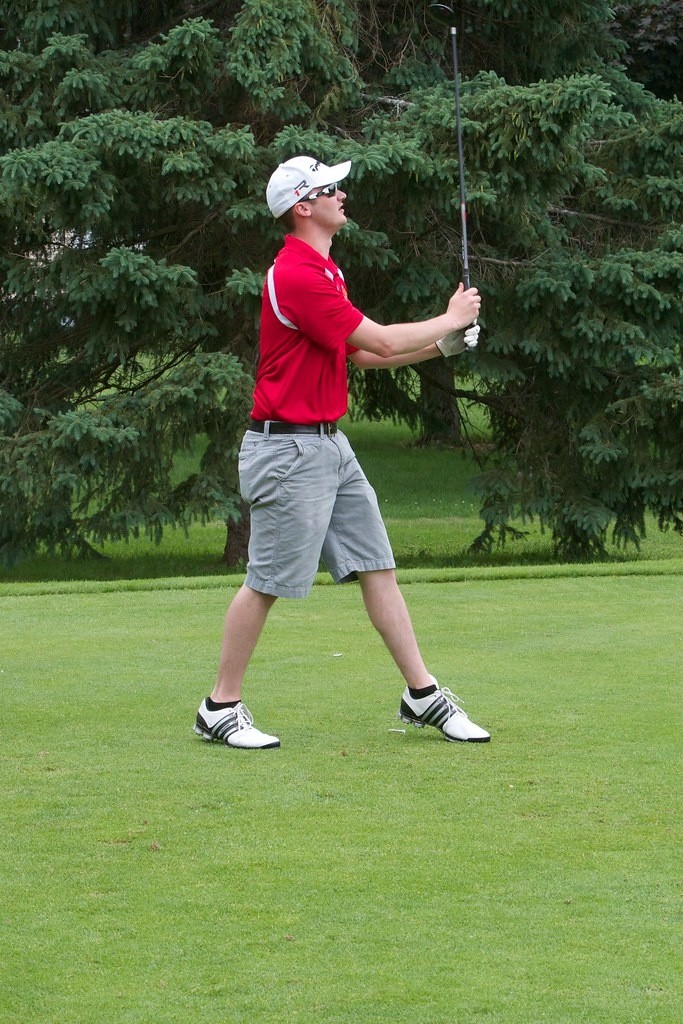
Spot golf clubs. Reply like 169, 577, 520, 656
426, 4, 476, 353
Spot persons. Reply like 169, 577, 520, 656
194, 156, 490, 749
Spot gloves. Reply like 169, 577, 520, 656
435, 318, 480, 356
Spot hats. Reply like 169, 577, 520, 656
266, 155, 352, 217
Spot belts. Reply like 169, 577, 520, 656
249, 420, 337, 434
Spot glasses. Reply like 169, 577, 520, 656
298, 183, 337, 202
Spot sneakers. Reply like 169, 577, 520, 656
397, 674, 491, 742
193, 698, 280, 749
